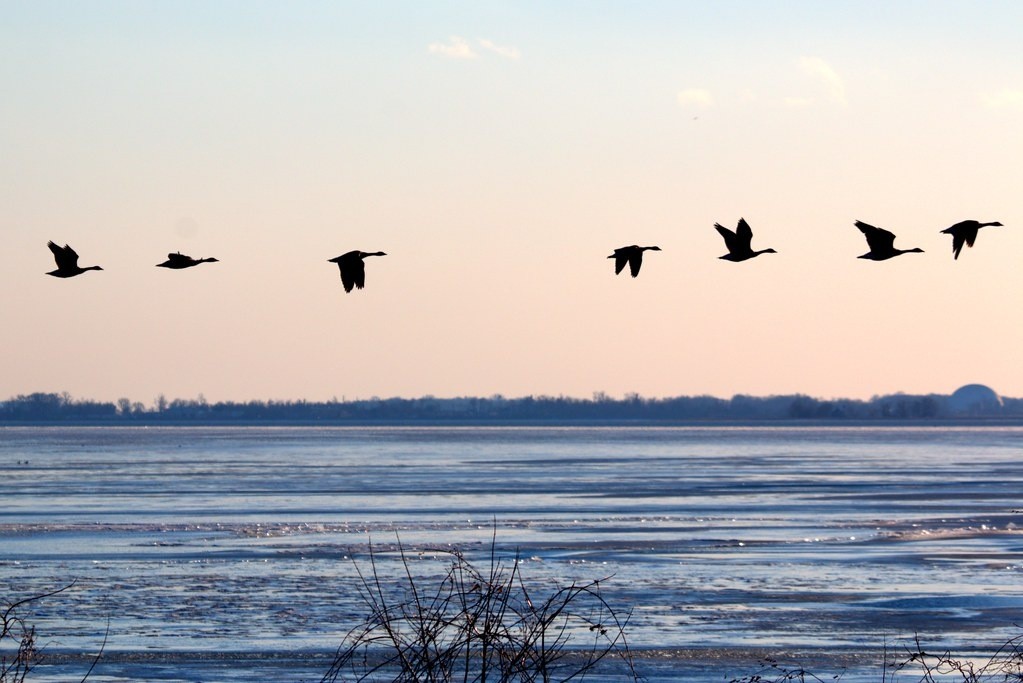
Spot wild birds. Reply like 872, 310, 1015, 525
607, 245, 662, 278
327, 250, 388, 293
940, 220, 1004, 260
45, 241, 104, 278
714, 217, 777, 262
854, 220, 926, 261
155, 251, 220, 269
